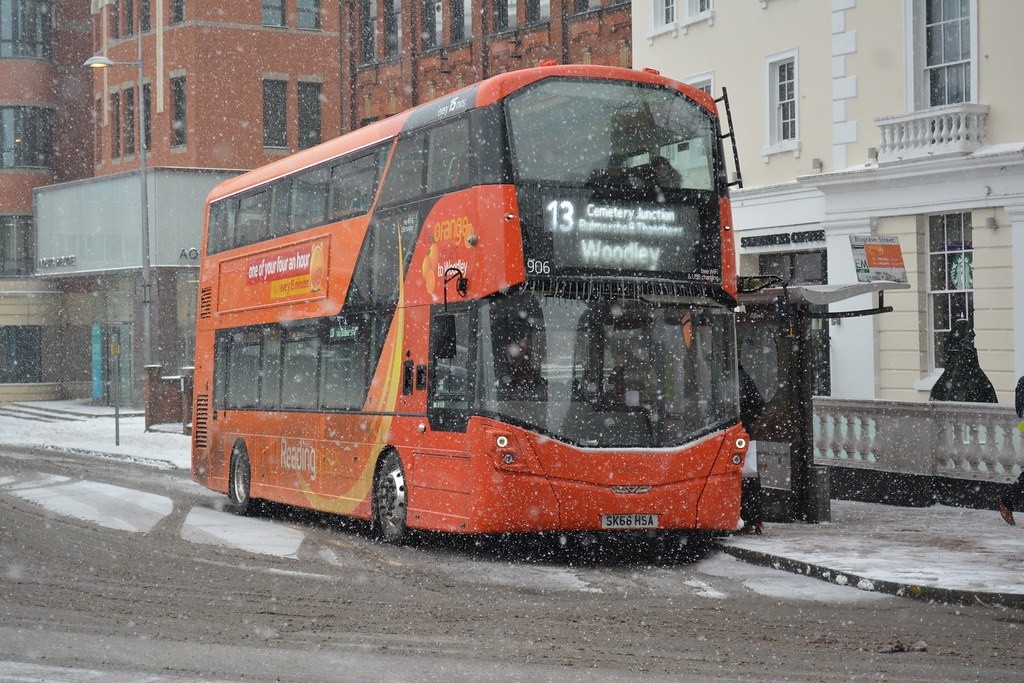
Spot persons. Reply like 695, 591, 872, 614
930, 321, 998, 403
738, 363, 764, 532
648, 156, 678, 187
583, 157, 633, 188
996, 377, 1024, 525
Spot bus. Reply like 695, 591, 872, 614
190, 58, 794, 547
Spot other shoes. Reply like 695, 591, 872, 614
735, 524, 763, 535
993, 495, 1015, 525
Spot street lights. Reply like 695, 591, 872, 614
83, 58, 168, 362
